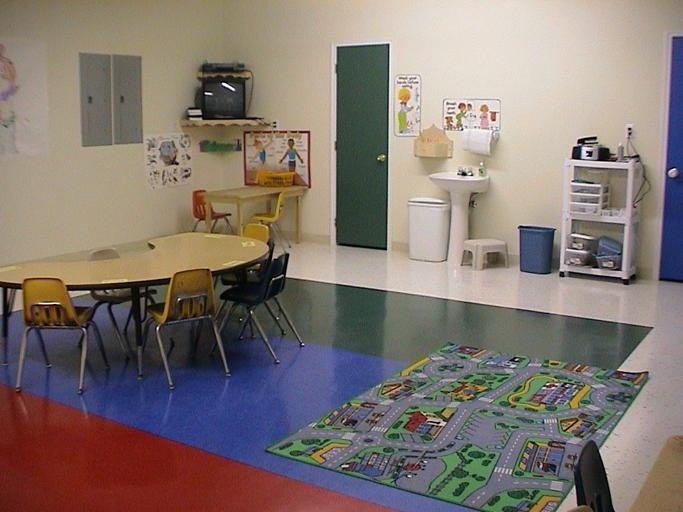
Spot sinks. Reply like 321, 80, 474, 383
429, 171, 490, 193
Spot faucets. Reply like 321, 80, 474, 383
459, 168, 466, 174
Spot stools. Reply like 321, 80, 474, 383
461, 238, 508, 272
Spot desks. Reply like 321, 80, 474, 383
0, 232, 270, 369
197, 185, 308, 245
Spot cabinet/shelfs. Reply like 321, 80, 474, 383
559, 155, 641, 285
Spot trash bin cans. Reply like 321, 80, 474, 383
517, 225, 557, 274
407, 197, 450, 262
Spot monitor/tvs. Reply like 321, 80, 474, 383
202, 81, 246, 119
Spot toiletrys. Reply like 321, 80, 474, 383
478, 162, 486, 176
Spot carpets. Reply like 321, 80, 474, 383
264, 343, 650, 512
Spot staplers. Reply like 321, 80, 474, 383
577, 136, 598, 145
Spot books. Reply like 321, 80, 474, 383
187, 106, 203, 119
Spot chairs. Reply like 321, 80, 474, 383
210, 253, 305, 364
140, 268, 231, 390
250, 189, 291, 253
564, 505, 593, 512
214, 242, 275, 319
243, 223, 271, 244
14, 277, 110, 395
77, 249, 158, 359
573, 439, 617, 512
191, 189, 236, 234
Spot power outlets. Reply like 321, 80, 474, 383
625, 123, 634, 138
